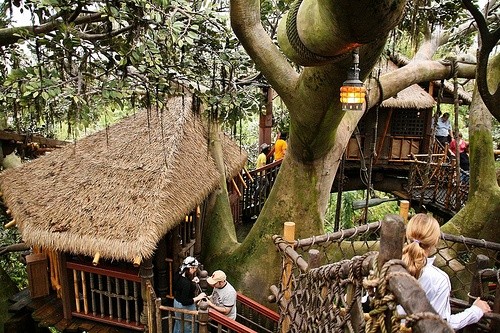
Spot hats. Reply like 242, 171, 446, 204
207, 270, 226, 284
260, 143, 270, 151
181, 256, 199, 271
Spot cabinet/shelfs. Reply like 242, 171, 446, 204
26, 256, 50, 300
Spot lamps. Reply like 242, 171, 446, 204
338, 43, 366, 110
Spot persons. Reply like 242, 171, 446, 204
251, 143, 270, 202
433, 112, 454, 159
453, 143, 470, 206
273, 130, 289, 163
430, 110, 442, 148
447, 131, 467, 182
173, 256, 206, 333
343, 213, 492, 333
197, 270, 237, 333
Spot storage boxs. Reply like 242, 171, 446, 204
387, 136, 423, 160
